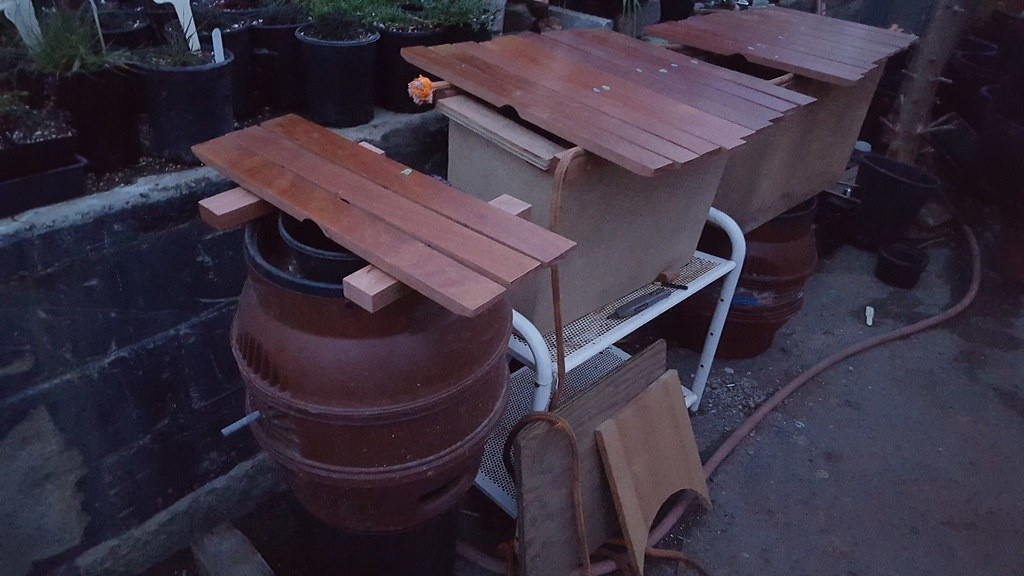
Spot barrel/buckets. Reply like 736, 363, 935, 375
841, 152, 941, 251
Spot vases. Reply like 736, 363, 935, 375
275, 213, 367, 284
874, 239, 930, 291
0, 153, 89, 219
843, 151, 943, 252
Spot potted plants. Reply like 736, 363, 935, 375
0, 0, 505, 175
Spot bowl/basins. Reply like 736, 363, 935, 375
875, 242, 929, 290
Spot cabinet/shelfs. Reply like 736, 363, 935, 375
474, 208, 746, 519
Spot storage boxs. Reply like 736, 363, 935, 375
433, 85, 730, 340
662, 42, 891, 236
